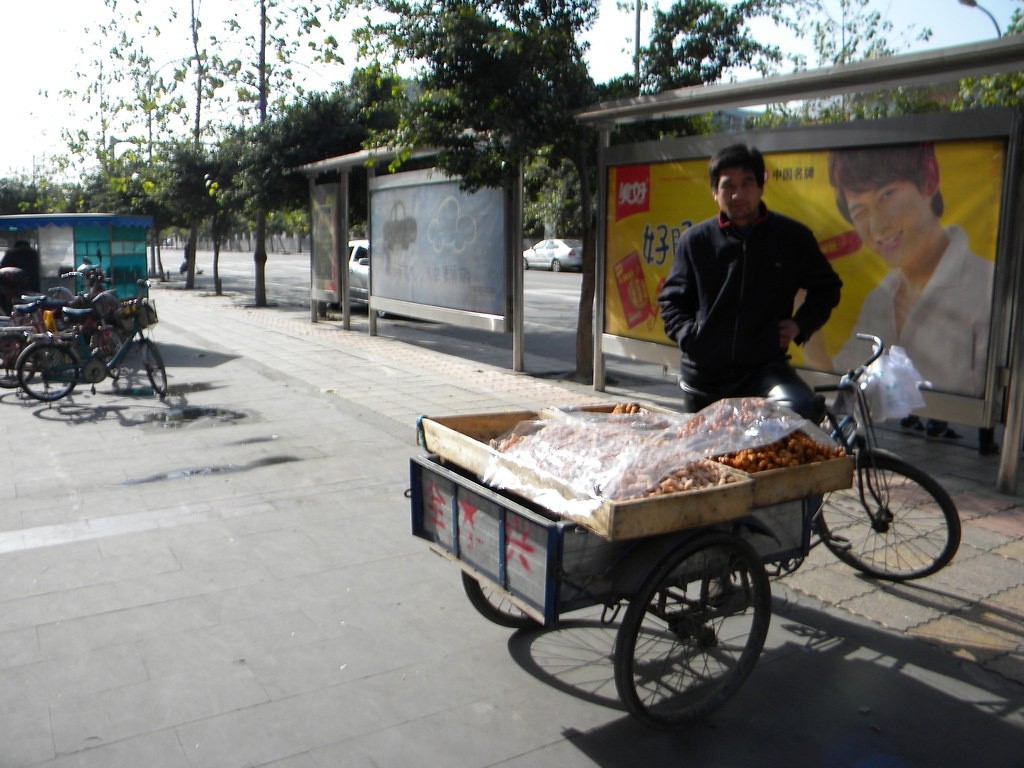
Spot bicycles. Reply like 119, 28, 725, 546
0, 247, 168, 410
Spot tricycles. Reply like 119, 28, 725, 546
404, 331, 964, 731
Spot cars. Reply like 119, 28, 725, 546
523, 238, 583, 272
326, 240, 388, 318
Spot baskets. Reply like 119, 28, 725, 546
113, 300, 159, 332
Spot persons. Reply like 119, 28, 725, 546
0, 238, 44, 315
825, 141, 994, 399
656, 136, 846, 605
178, 237, 192, 276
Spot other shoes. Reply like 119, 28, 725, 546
900, 418, 925, 432
926, 428, 964, 445
706, 579, 731, 598
979, 443, 999, 454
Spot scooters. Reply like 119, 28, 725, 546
0, 213, 158, 344
